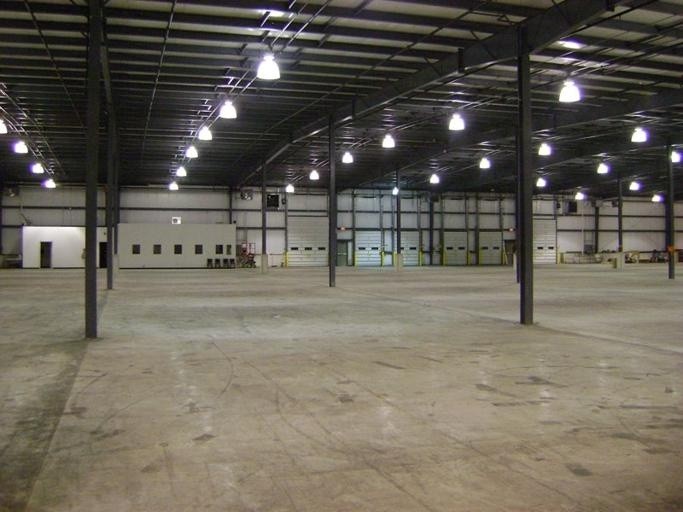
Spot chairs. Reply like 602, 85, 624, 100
205, 257, 238, 269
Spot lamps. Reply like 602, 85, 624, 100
0, 116, 57, 189
